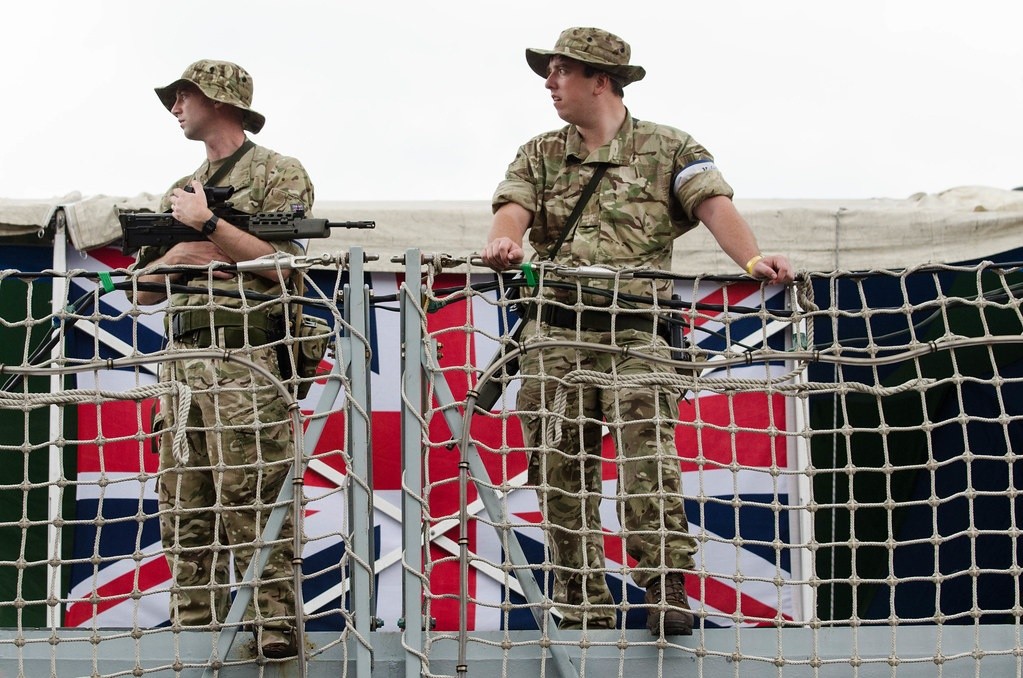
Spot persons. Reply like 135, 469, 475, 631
124, 59, 315, 656
482, 28, 795, 635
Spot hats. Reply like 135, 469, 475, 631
153, 59, 266, 136
524, 27, 646, 87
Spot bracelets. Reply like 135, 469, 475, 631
745, 256, 761, 275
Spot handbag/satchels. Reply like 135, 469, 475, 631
281, 311, 333, 402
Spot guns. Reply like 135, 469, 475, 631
115, 185, 376, 257
445, 320, 528, 450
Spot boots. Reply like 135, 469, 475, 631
643, 570, 695, 636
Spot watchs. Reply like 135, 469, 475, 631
200, 215, 219, 237
